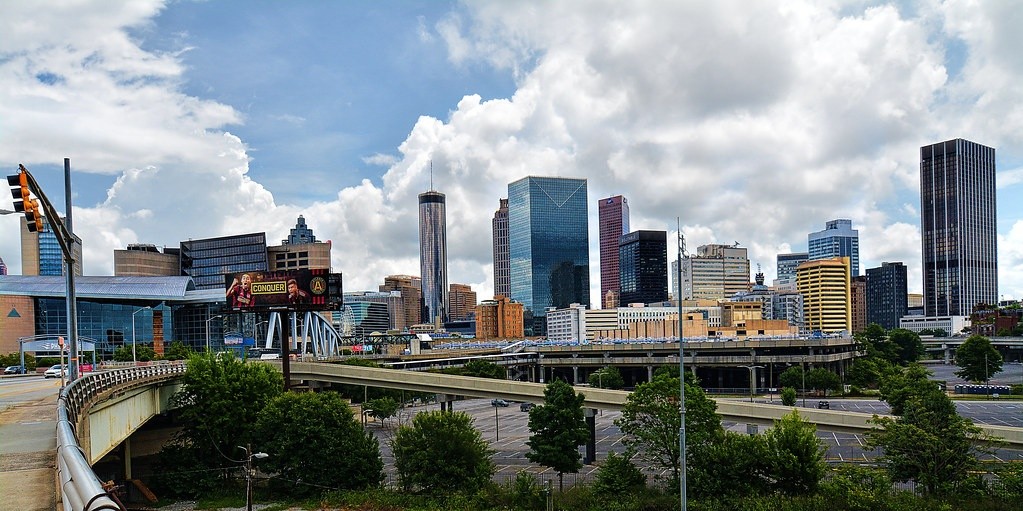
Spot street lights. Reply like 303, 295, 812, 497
132, 306, 150, 362
289, 317, 303, 349
206, 315, 222, 354
353, 327, 365, 357
677, 215, 689, 511
255, 321, 269, 348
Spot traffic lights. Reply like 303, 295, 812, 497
6, 172, 33, 213
26, 198, 43, 233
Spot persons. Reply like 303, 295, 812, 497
100, 359, 104, 371
226, 273, 256, 310
286, 279, 310, 304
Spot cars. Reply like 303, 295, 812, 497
240, 331, 838, 368
519, 403, 536, 413
44, 364, 69, 379
818, 400, 830, 410
491, 399, 509, 408
80, 362, 93, 373
3, 366, 27, 375
